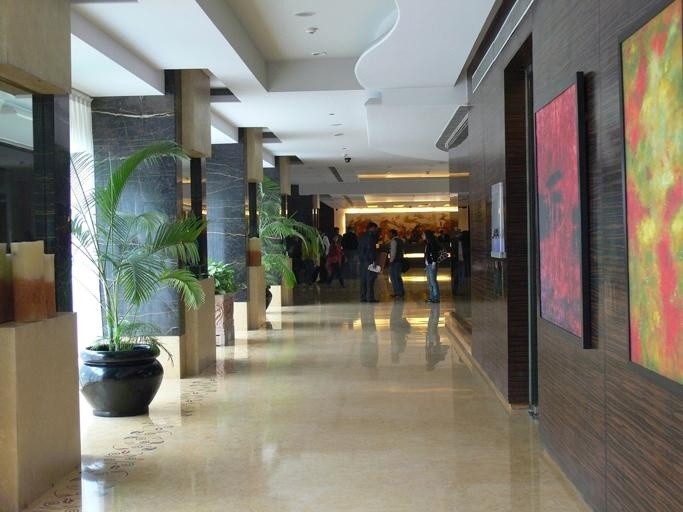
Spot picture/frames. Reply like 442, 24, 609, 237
532, 67, 594, 351
617, 0, 682, 407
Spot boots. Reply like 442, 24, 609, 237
339, 279, 347, 288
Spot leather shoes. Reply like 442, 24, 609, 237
424, 298, 440, 304
390, 293, 405, 300
359, 299, 380, 303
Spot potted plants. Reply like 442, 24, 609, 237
256, 173, 328, 315
203, 255, 247, 349
35, 126, 216, 420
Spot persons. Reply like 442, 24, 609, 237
356, 303, 445, 380
313, 223, 461, 304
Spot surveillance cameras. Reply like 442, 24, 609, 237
344, 158, 351, 163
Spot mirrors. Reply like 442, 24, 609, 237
0, 73, 38, 327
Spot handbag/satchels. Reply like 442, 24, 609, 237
400, 259, 410, 273
367, 263, 382, 273
342, 255, 347, 263
429, 240, 449, 264
